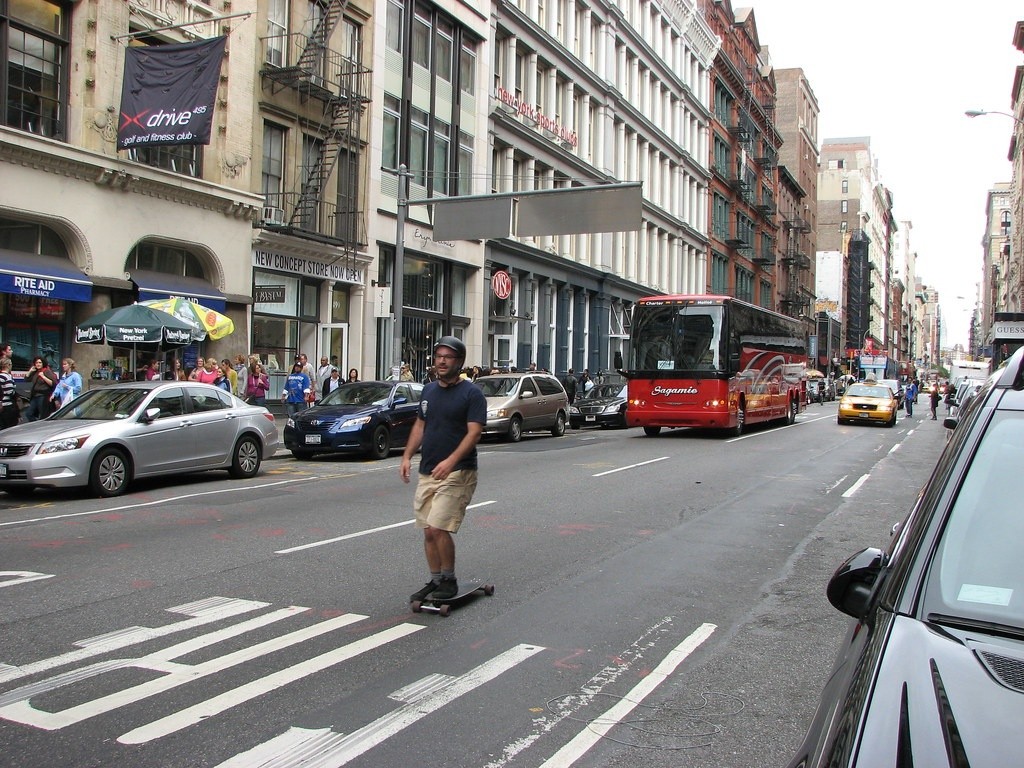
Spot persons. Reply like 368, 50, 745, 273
399, 362, 604, 407
281, 354, 363, 418
929, 383, 938, 420
0, 342, 19, 430
24, 356, 82, 422
817, 377, 855, 403
904, 376, 920, 416
136, 349, 270, 407
400, 336, 488, 606
943, 381, 959, 410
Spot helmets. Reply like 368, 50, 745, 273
433, 336, 466, 370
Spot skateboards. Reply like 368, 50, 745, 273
411, 576, 497, 617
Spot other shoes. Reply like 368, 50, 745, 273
931, 417, 936, 420
905, 414, 912, 417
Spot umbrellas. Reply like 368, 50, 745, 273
75, 297, 235, 382
806, 369, 824, 379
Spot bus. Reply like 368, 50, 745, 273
614, 295, 808, 437
854, 354, 897, 382
899, 361, 910, 385
920, 371, 939, 393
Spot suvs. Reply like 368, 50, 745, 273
472, 371, 570, 443
783, 347, 1024, 768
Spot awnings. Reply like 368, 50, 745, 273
0, 247, 93, 303
126, 268, 226, 315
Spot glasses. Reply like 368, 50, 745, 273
433, 352, 462, 360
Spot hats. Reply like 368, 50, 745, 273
294, 361, 303, 368
331, 367, 339, 372
294, 354, 300, 362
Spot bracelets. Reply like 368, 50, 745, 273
282, 396, 285, 397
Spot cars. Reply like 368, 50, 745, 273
568, 383, 630, 431
939, 384, 948, 394
878, 380, 905, 410
0, 381, 278, 497
283, 380, 426, 460
943, 360, 989, 441
837, 382, 897, 428
806, 378, 835, 404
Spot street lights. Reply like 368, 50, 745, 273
862, 327, 883, 346
958, 296, 995, 374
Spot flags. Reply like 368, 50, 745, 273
116, 34, 228, 154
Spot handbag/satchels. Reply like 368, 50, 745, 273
584, 380, 594, 391
59, 388, 75, 414
937, 395, 942, 401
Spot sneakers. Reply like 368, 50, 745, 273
410, 578, 439, 602
432, 576, 458, 598
